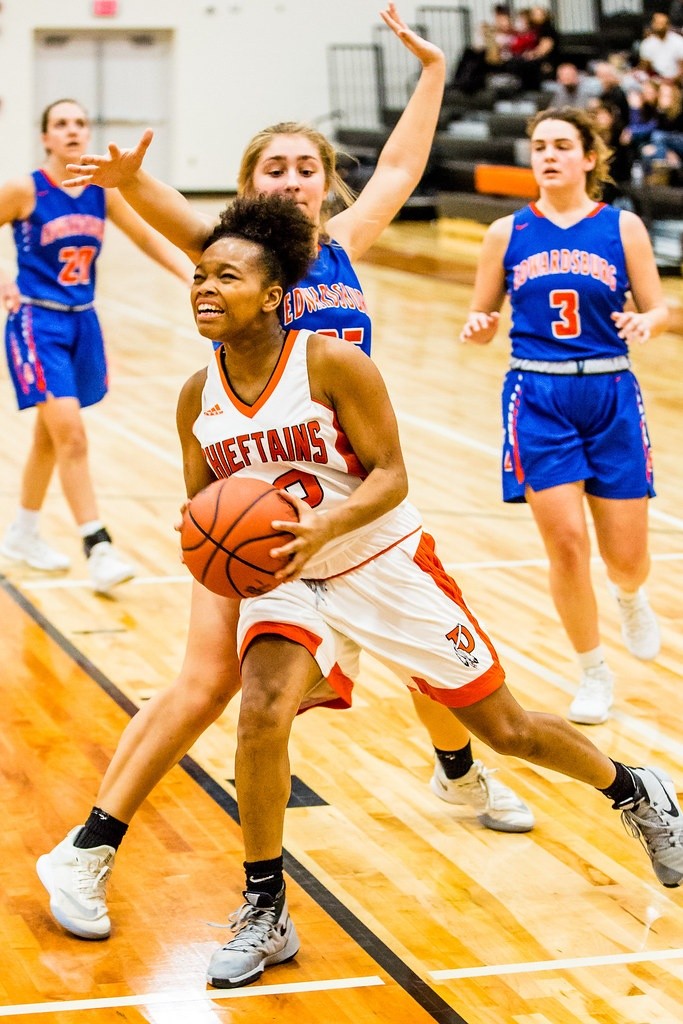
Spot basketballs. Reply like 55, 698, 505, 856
180, 475, 300, 599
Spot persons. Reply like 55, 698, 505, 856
36, 3, 534, 939
458, 106, 671, 725
0, 100, 193, 585
175, 193, 682, 988
445, 0, 683, 224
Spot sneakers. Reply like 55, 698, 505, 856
4, 525, 68, 570
37, 824, 117, 940
205, 879, 301, 988
612, 764, 683, 888
607, 578, 661, 660
431, 761, 536, 832
86, 540, 133, 589
567, 669, 614, 724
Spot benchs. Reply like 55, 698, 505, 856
327, 0, 683, 278
